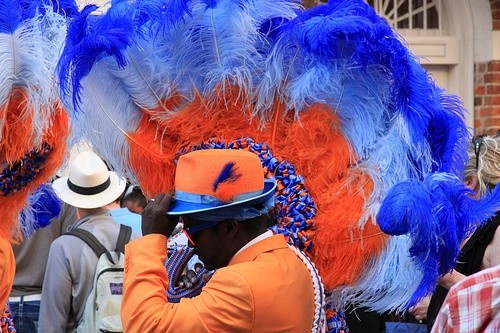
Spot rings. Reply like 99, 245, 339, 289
150, 198, 155, 201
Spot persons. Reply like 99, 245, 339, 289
0, 133, 500, 333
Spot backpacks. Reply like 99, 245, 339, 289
60, 224, 132, 333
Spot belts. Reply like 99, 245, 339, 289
8, 293, 43, 302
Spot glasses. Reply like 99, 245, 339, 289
183, 227, 195, 245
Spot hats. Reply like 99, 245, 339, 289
52, 151, 126, 208
166, 149, 276, 214
124, 183, 142, 198
